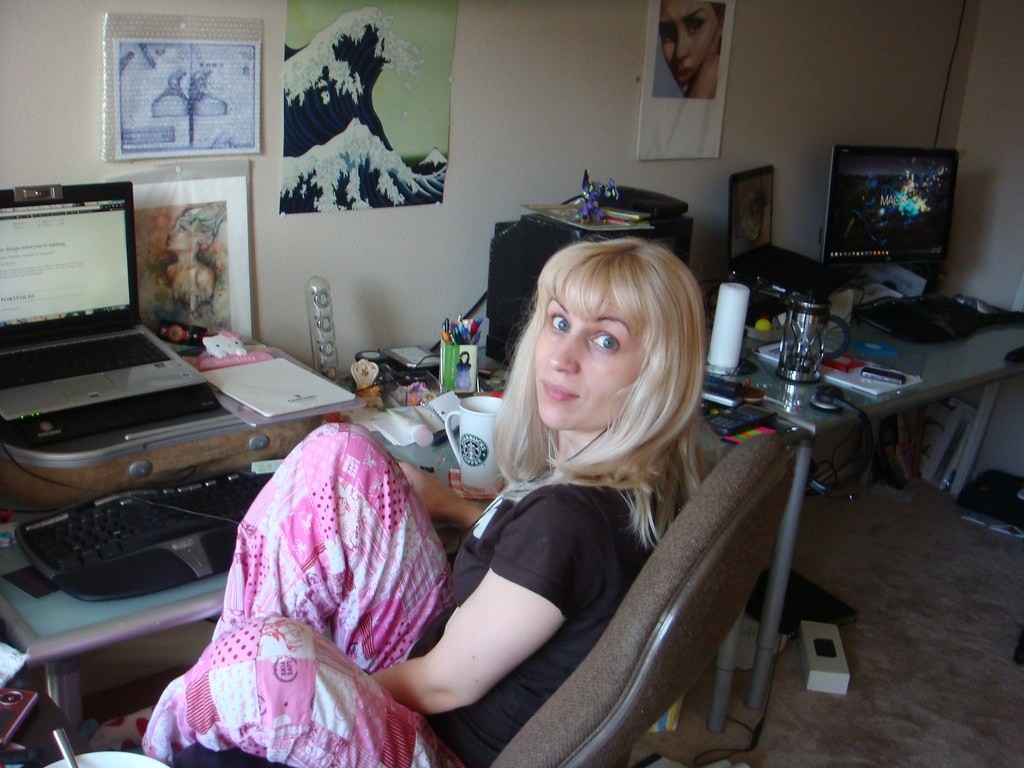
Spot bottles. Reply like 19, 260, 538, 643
355, 350, 386, 394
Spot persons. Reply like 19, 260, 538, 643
140, 234, 727, 767
659, 0, 726, 103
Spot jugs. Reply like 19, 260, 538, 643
774, 291, 852, 384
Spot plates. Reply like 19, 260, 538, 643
42, 751, 171, 768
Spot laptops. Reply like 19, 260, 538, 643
0, 180, 206, 422
728, 164, 825, 294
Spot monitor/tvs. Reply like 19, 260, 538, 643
820, 143, 960, 268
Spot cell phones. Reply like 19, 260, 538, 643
860, 367, 907, 386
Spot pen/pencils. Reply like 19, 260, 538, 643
432, 425, 461, 446
439, 314, 484, 345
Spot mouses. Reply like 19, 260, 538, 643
956, 295, 999, 316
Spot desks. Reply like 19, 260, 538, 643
1, 372, 805, 734
687, 290, 1023, 706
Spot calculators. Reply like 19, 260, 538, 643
706, 402, 778, 435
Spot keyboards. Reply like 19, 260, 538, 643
853, 290, 976, 348
14, 473, 277, 602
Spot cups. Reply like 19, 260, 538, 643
444, 396, 505, 495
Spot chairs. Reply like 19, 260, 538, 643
487, 431, 799, 766
